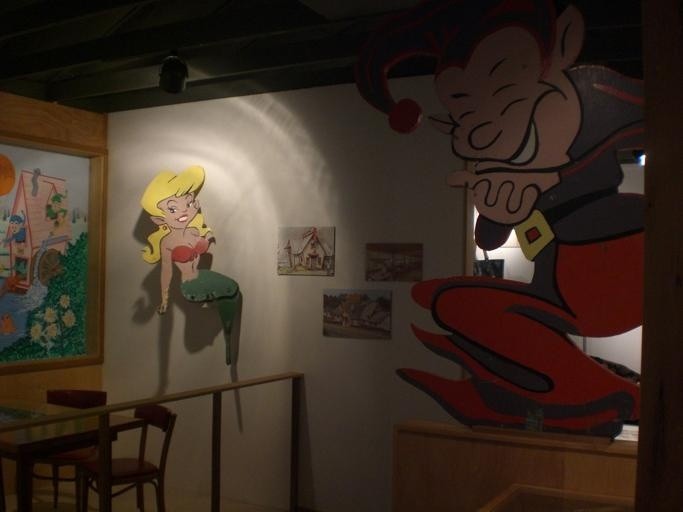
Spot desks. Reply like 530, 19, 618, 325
0, 398, 144, 512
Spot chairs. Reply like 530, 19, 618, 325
32, 389, 107, 512
76, 405, 177, 512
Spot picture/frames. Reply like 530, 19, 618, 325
0, 131, 108, 377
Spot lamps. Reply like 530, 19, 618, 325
160, 56, 187, 93
